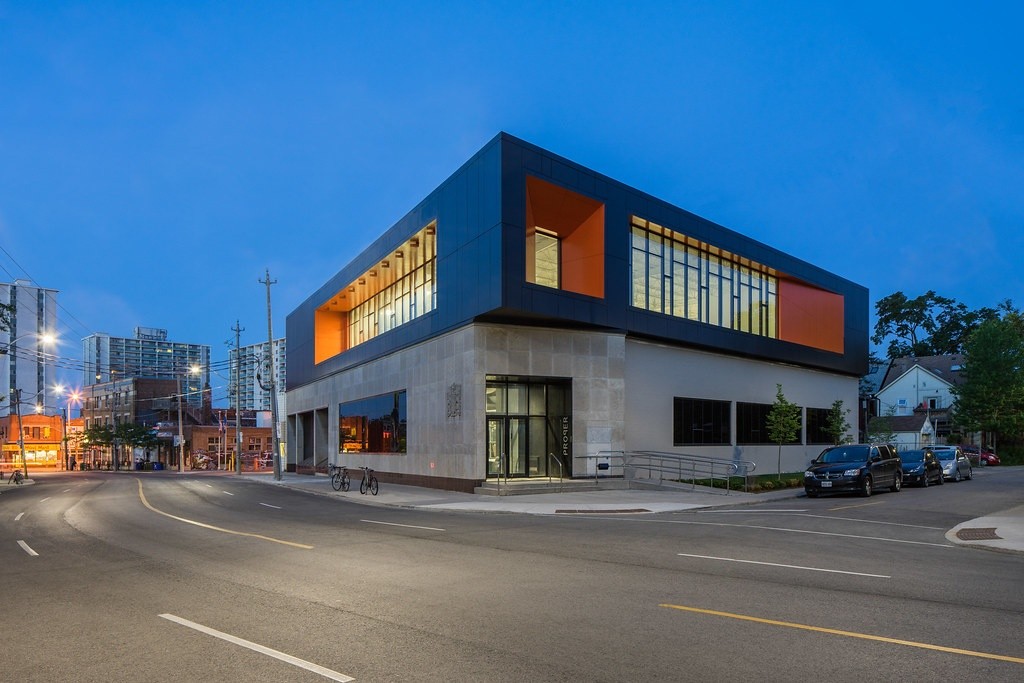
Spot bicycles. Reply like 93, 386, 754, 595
7, 470, 24, 484
329, 465, 351, 492
358, 466, 379, 496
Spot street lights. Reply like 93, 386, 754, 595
112, 370, 121, 472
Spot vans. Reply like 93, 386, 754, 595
804, 443, 904, 499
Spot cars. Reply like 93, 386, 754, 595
927, 449, 973, 482
897, 449, 945, 487
959, 445, 1001, 465
